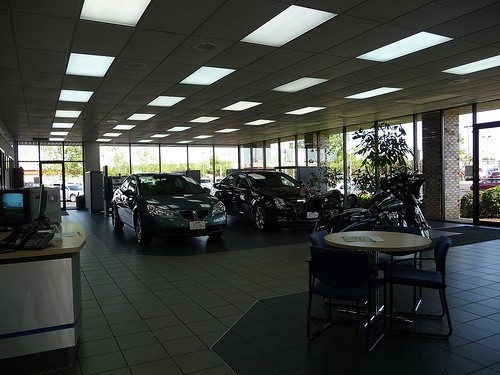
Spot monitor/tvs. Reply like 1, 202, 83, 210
38, 184, 48, 221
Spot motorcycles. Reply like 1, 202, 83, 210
304, 169, 433, 238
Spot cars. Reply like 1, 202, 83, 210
469, 178, 500, 191
110, 172, 228, 246
200, 178, 216, 190
489, 171, 500, 178
60, 184, 84, 202
213, 170, 313, 230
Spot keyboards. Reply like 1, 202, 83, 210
24, 231, 55, 249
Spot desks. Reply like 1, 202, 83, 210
324, 232, 431, 334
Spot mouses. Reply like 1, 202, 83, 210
1, 241, 11, 250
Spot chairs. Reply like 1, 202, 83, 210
305, 224, 453, 352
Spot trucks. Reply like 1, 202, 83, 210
465, 166, 486, 181
